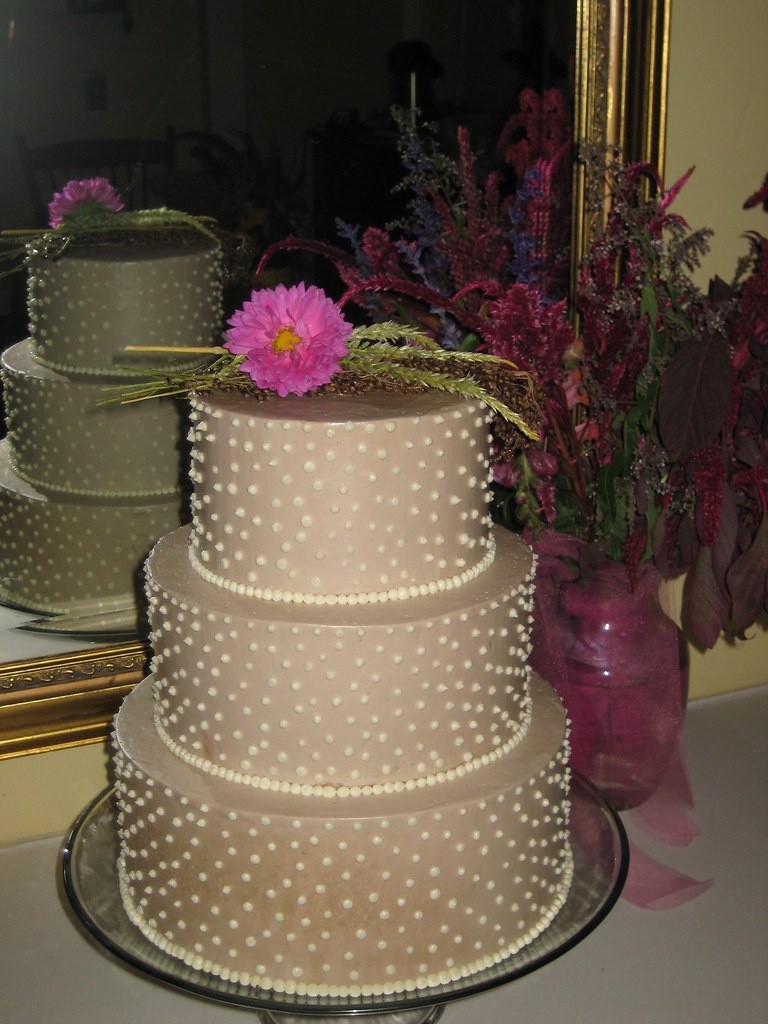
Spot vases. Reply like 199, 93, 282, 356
541, 568, 688, 812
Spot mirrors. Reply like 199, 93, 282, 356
0, 0, 669, 761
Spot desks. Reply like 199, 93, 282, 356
0, 682, 768, 1024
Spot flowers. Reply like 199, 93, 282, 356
251, 80, 767, 646
1, 177, 220, 275
92, 284, 541, 443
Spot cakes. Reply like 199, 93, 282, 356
0, 227, 224, 608
108, 384, 574, 997
